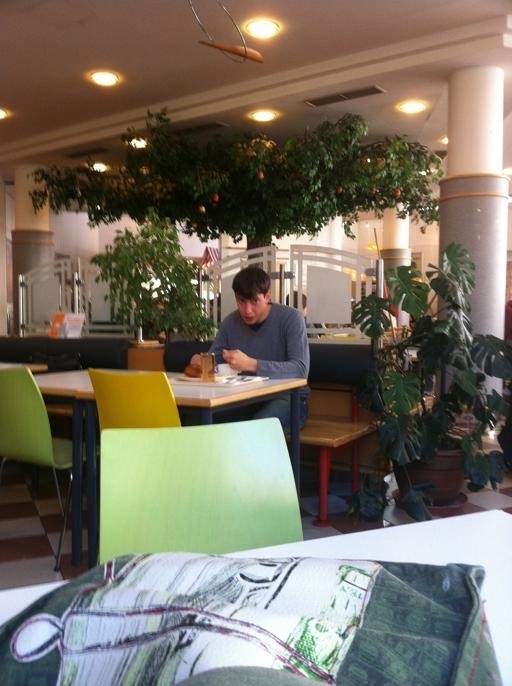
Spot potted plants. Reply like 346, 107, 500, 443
352, 262, 512, 521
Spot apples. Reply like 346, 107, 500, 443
258, 171, 265, 181
198, 206, 206, 215
394, 189, 401, 200
213, 194, 219, 202
337, 186, 343, 194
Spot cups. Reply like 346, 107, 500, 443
200, 351, 216, 385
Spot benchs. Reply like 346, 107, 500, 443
295, 346, 380, 527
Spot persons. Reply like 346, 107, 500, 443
190, 265, 310, 440
284, 290, 308, 318
